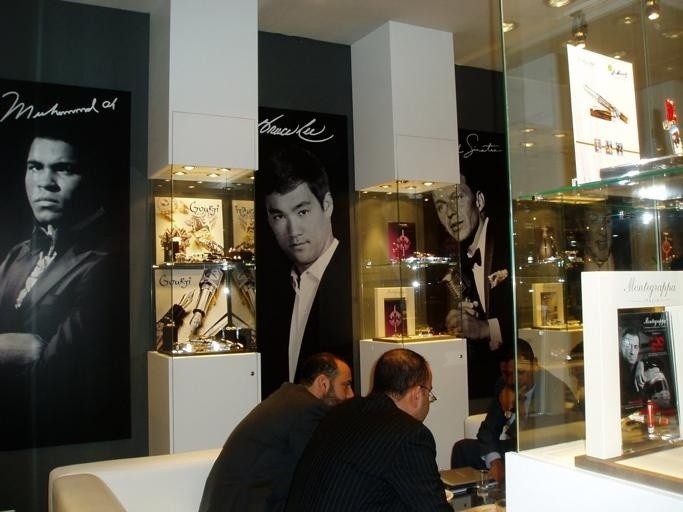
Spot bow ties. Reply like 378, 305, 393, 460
467, 248, 482, 269
28, 229, 62, 255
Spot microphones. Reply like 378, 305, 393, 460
190, 269, 224, 332
232, 267, 256, 319
442, 269, 473, 303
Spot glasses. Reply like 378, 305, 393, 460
413, 383, 437, 405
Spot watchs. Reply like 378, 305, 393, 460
504, 408, 513, 420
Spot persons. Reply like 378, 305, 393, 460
450, 338, 574, 469
619, 326, 664, 404
0, 127, 130, 447
569, 341, 585, 412
432, 173, 514, 354
562, 202, 632, 321
264, 168, 354, 385
287, 349, 504, 512
198, 354, 354, 512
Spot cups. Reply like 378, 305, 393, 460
474, 470, 488, 497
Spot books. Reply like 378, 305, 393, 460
438, 467, 496, 494
